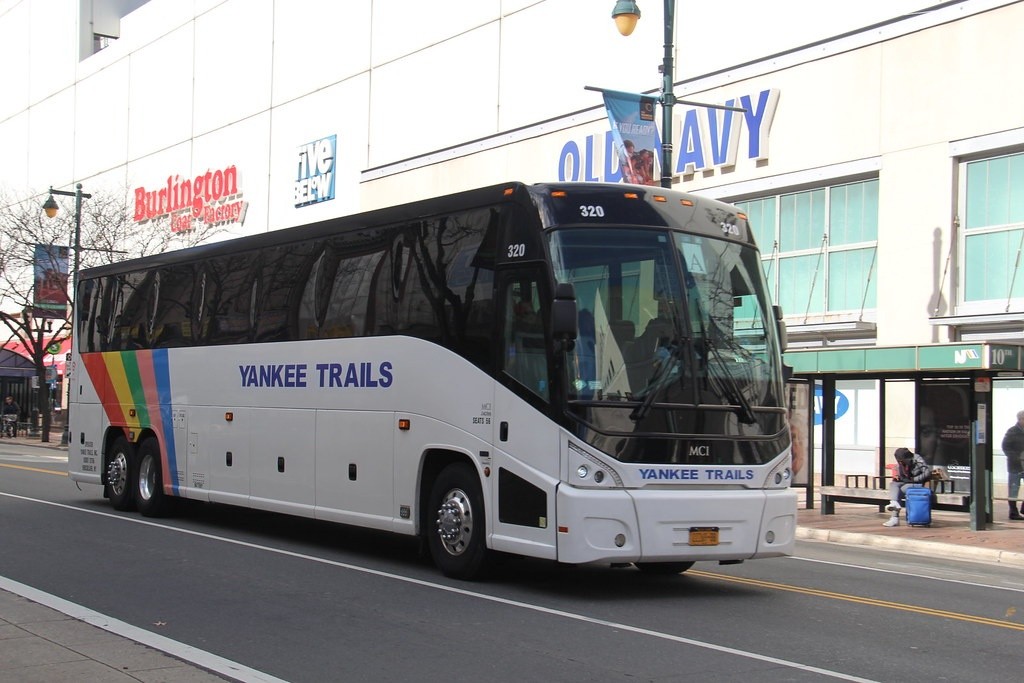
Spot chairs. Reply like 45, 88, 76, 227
515, 306, 639, 394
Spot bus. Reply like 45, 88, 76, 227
65, 177, 801, 583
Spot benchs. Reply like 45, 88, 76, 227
820, 475, 970, 513
4, 422, 31, 436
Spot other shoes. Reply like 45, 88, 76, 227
882, 516, 900, 527
885, 501, 902, 512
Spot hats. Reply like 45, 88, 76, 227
1016, 410, 1024, 419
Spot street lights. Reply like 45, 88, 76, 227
43, 184, 127, 298
612, 0, 677, 190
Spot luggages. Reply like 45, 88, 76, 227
906, 482, 932, 527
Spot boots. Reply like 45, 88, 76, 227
1020, 501, 1024, 514
1008, 501, 1024, 520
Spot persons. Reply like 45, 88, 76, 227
3, 396, 20, 437
622, 140, 653, 185
1001, 411, 1024, 520
919, 400, 939, 465
624, 316, 714, 403
883, 447, 932, 526
